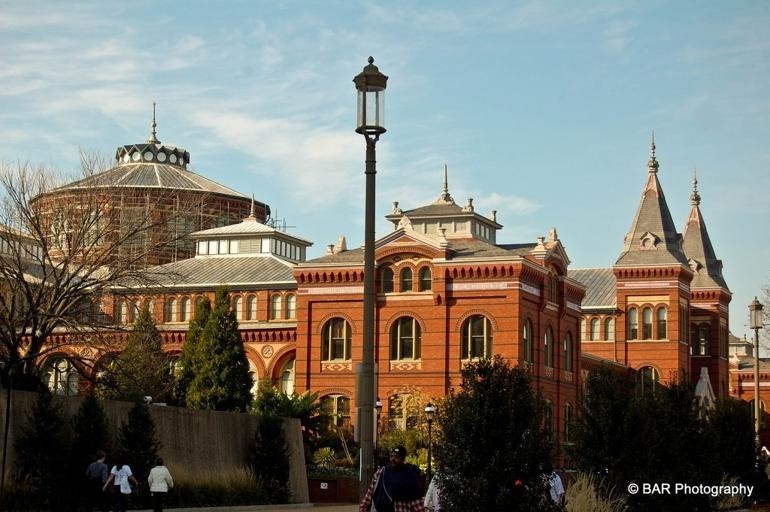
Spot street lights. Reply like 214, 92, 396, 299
425, 402, 435, 483
353, 57, 388, 503
748, 296, 764, 472
374, 398, 382, 473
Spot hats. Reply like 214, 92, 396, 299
390, 445, 406, 457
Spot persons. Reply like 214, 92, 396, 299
359, 444, 427, 512
423, 462, 465, 512
85, 448, 112, 511
542, 463, 566, 512
101, 455, 140, 512
147, 456, 176, 511
